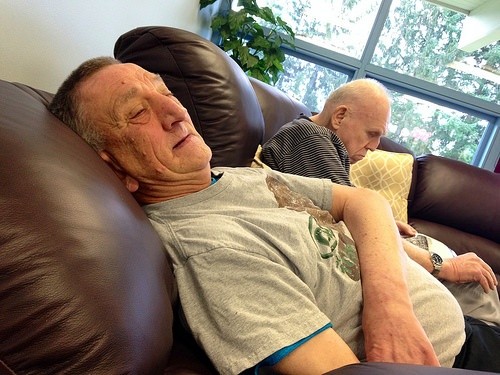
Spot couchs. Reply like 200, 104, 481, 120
0, 28, 500, 375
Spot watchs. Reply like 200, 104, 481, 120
430, 251, 444, 277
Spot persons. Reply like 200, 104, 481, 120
259, 79, 500, 328
49, 57, 500, 375
